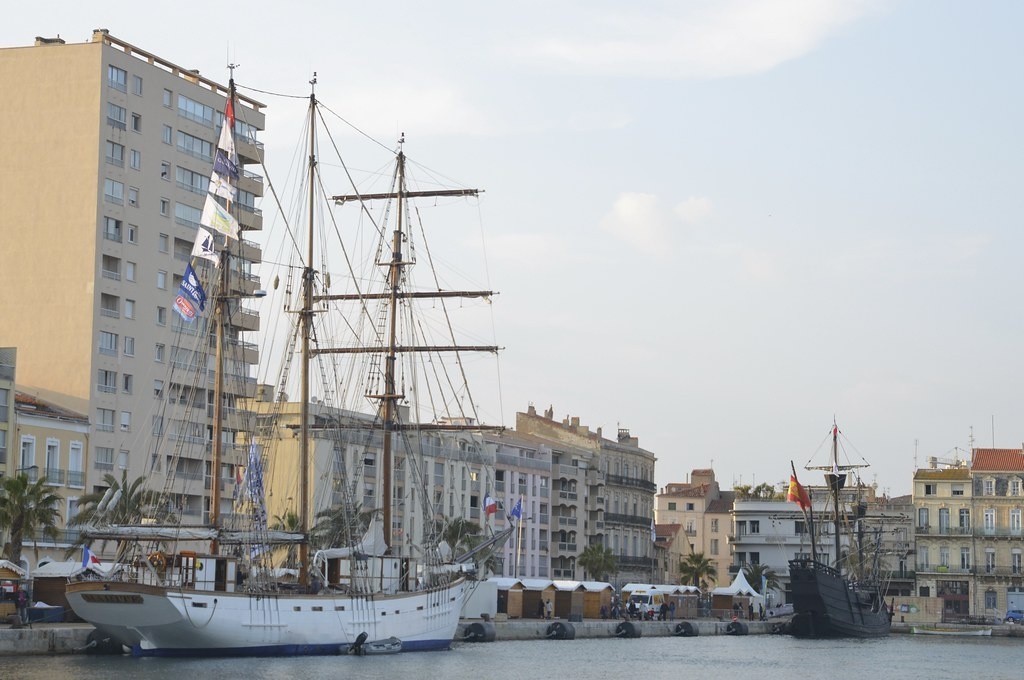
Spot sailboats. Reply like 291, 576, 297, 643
64, 39, 515, 659
768, 414, 909, 639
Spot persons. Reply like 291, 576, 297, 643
15, 586, 29, 625
538, 599, 765, 621
545, 599, 553, 620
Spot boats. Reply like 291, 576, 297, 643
910, 626, 991, 636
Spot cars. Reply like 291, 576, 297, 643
1006, 609, 1024, 622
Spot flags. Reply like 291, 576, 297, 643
511, 498, 521, 519
192, 228, 220, 269
173, 99, 240, 322
482, 492, 497, 513
786, 475, 811, 508
82, 545, 100, 567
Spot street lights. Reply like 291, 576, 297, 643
516, 517, 534, 579
12, 465, 38, 522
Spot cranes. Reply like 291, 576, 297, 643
929, 446, 971, 470
